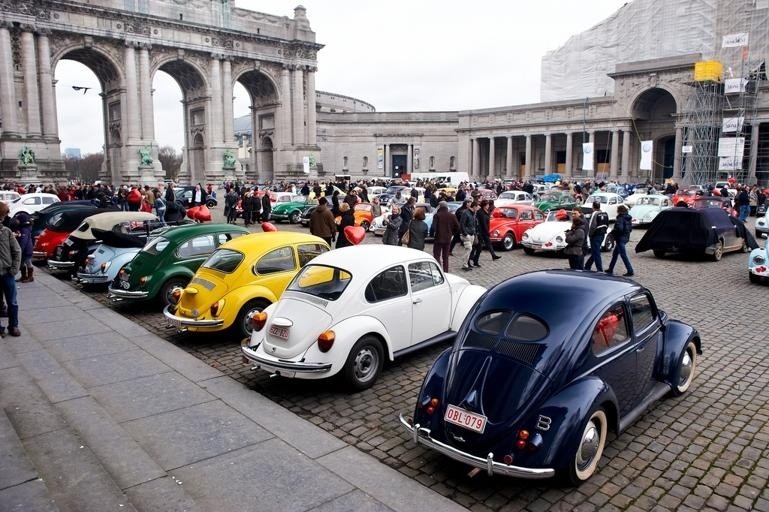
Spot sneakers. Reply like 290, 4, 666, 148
492, 255, 501, 260
473, 263, 481, 267
0, 325, 7, 338
7, 326, 21, 337
623, 272, 634, 277
604, 269, 613, 274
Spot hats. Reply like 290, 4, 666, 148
464, 198, 472, 202
143, 185, 151, 188
439, 200, 448, 208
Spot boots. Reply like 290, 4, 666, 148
22, 267, 34, 283
14, 265, 28, 283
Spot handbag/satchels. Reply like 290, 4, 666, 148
401, 229, 410, 244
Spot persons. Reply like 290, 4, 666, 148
556, 180, 678, 276
191, 184, 206, 207
16, 215, 33, 282
709, 180, 769, 222
0, 181, 186, 221
208, 184, 212, 195
223, 177, 544, 273
0, 202, 22, 336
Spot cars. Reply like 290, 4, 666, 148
107, 223, 252, 308
153, 183, 219, 208
242, 244, 488, 388
163, 231, 334, 338
0, 186, 154, 287
400, 268, 705, 488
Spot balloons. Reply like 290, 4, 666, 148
595, 314, 617, 346
730, 178, 735, 183
186, 206, 212, 223
